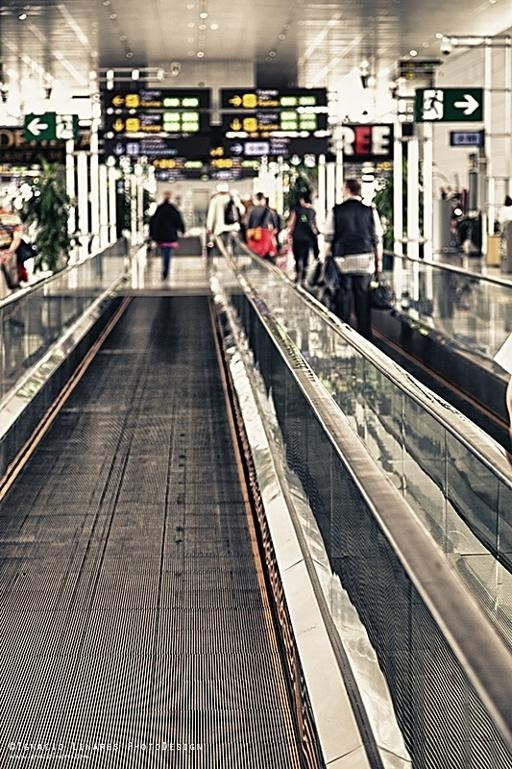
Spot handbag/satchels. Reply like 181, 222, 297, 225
368, 273, 395, 310
224, 201, 239, 224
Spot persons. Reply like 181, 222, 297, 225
492, 330, 511, 419
206, 178, 383, 339
149, 191, 185, 281
0, 205, 24, 300
